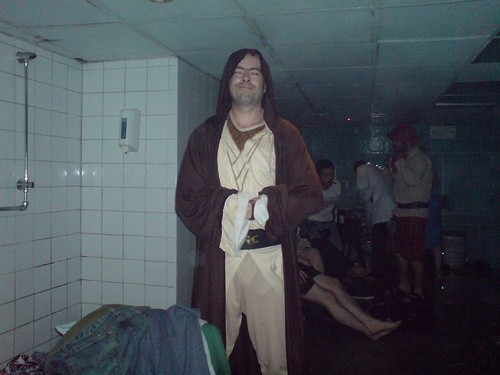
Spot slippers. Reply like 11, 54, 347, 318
401, 293, 425, 304
385, 286, 410, 296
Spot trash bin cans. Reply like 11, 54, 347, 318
440, 228, 468, 276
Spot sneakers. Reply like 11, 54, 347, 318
352, 290, 375, 300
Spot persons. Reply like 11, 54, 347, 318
384, 125, 433, 305
299, 160, 342, 249
297, 261, 402, 341
295, 224, 377, 302
353, 160, 397, 276
416, 146, 446, 284
176, 49, 323, 375
